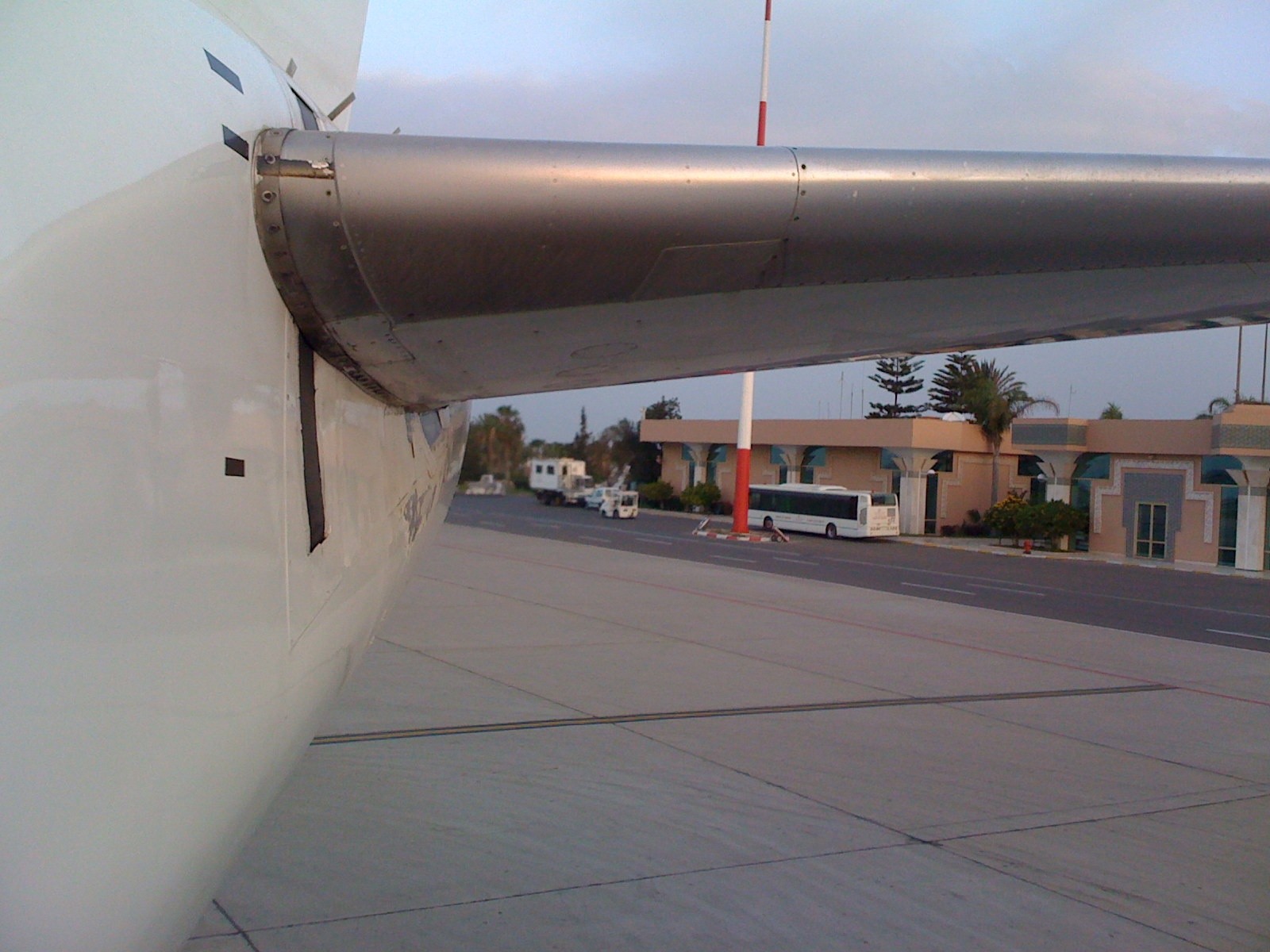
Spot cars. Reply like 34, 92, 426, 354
583, 487, 639, 519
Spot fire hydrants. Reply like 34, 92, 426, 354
1023, 539, 1031, 554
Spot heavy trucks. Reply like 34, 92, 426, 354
528, 457, 594, 509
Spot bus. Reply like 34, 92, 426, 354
747, 484, 900, 539
